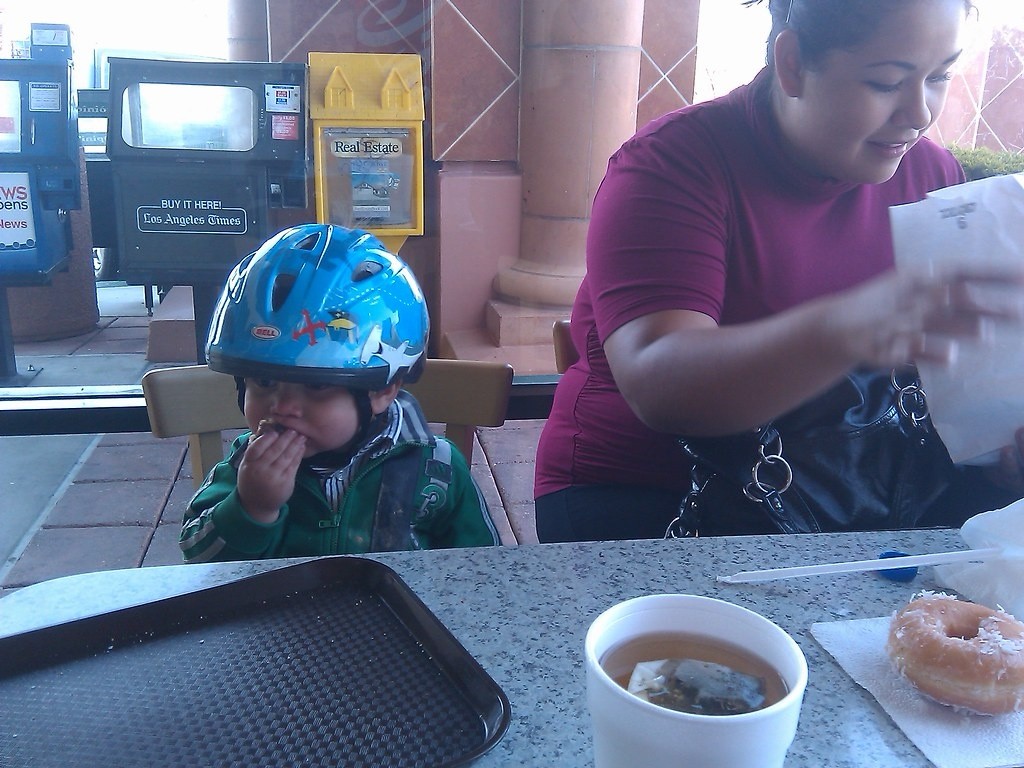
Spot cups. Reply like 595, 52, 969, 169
585, 593, 807, 768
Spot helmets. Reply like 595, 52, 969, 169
202, 221, 431, 390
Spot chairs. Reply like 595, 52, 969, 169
140, 360, 514, 490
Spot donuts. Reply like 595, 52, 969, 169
885, 590, 1024, 717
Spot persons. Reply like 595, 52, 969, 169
177, 222, 502, 563
535, 0, 1024, 544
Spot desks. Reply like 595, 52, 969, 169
1, 529, 1024, 768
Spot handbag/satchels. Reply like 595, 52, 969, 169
663, 362, 955, 538
